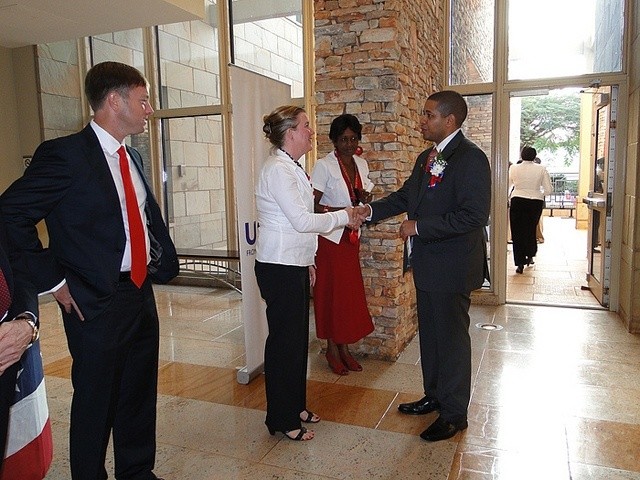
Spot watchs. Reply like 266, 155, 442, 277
21, 316, 39, 344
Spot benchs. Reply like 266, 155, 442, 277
174, 248, 242, 296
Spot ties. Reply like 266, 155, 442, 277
116, 145, 148, 290
426, 149, 437, 174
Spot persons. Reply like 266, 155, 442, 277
0, 240, 40, 480
347, 90, 492, 442
253, 106, 365, 441
308, 114, 373, 376
506, 146, 552, 272
1, 60, 179, 479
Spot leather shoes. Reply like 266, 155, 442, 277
420, 416, 468, 441
397, 395, 439, 415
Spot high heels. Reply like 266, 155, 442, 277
339, 349, 362, 371
299, 408, 321, 423
516, 264, 523, 274
326, 351, 349, 376
527, 261, 535, 266
264, 421, 315, 440
